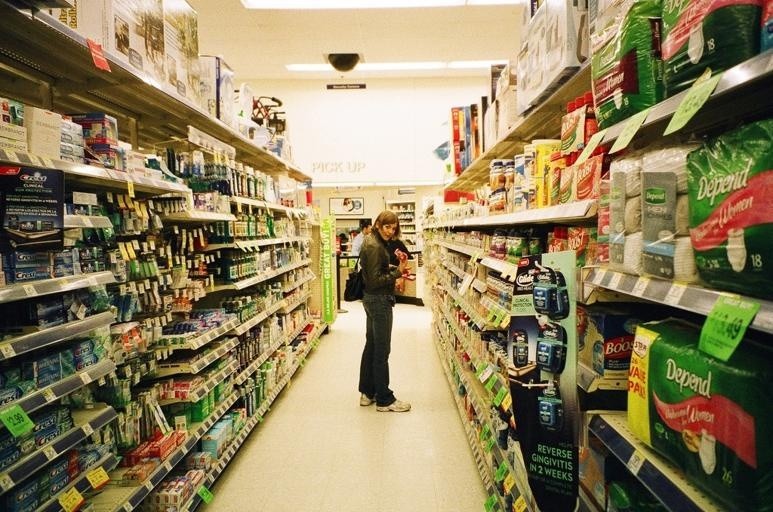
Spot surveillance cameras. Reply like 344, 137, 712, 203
327, 54, 362, 73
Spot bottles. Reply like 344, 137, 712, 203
218, 161, 279, 416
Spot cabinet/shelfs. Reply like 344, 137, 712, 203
423, 45, 772, 512
0, 1, 330, 511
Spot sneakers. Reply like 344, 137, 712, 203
376, 400, 410, 411
360, 393, 376, 405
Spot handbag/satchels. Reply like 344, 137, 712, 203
344, 269, 364, 301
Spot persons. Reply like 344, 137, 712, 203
384, 234, 415, 305
358, 211, 411, 412
351, 220, 372, 304
